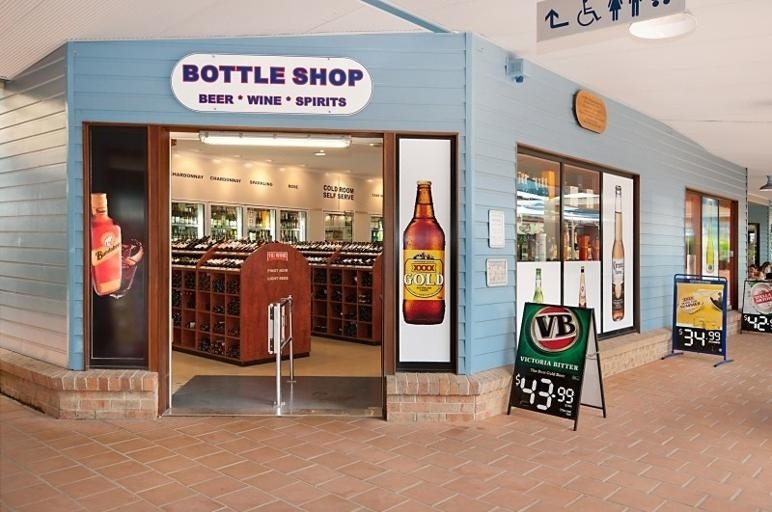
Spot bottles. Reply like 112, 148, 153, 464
90, 193, 124, 297
517, 169, 600, 261
402, 180, 446, 325
578, 266, 586, 309
706, 201, 714, 273
171, 202, 384, 360
610, 184, 626, 322
684, 198, 697, 280
531, 267, 543, 303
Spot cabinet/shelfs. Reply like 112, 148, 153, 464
168, 237, 384, 367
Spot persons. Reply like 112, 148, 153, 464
758, 262, 770, 273
748, 264, 763, 280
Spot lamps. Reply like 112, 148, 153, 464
759, 175, 772, 193
